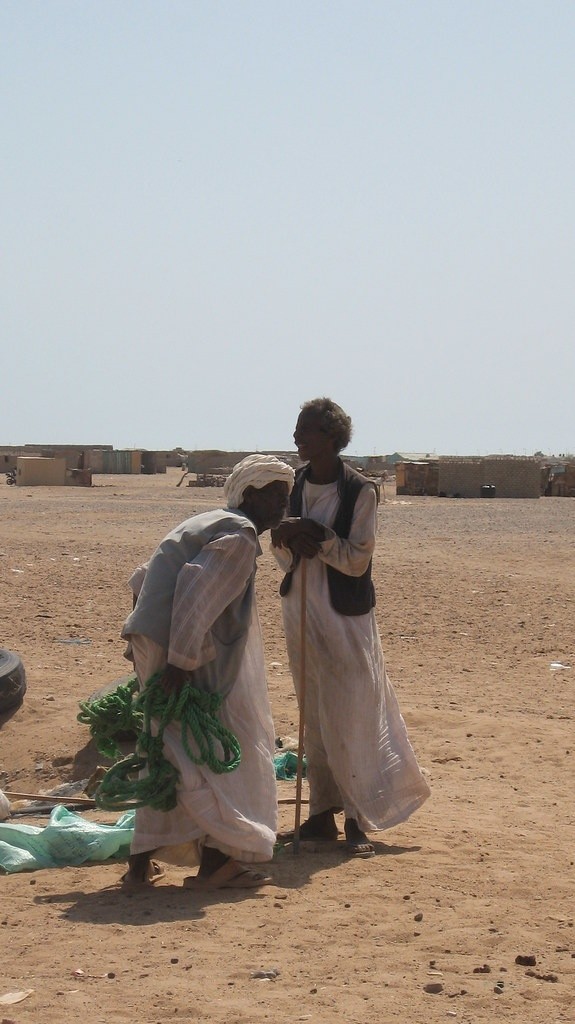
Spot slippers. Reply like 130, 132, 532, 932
347, 836, 375, 859
275, 819, 339, 839
184, 856, 274, 888
120, 860, 166, 886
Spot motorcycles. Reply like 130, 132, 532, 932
5, 472, 16, 485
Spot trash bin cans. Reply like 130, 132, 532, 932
480, 484, 496, 498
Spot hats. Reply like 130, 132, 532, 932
223, 454, 295, 509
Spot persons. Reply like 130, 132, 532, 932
268, 397, 431, 856
121, 454, 296, 888
